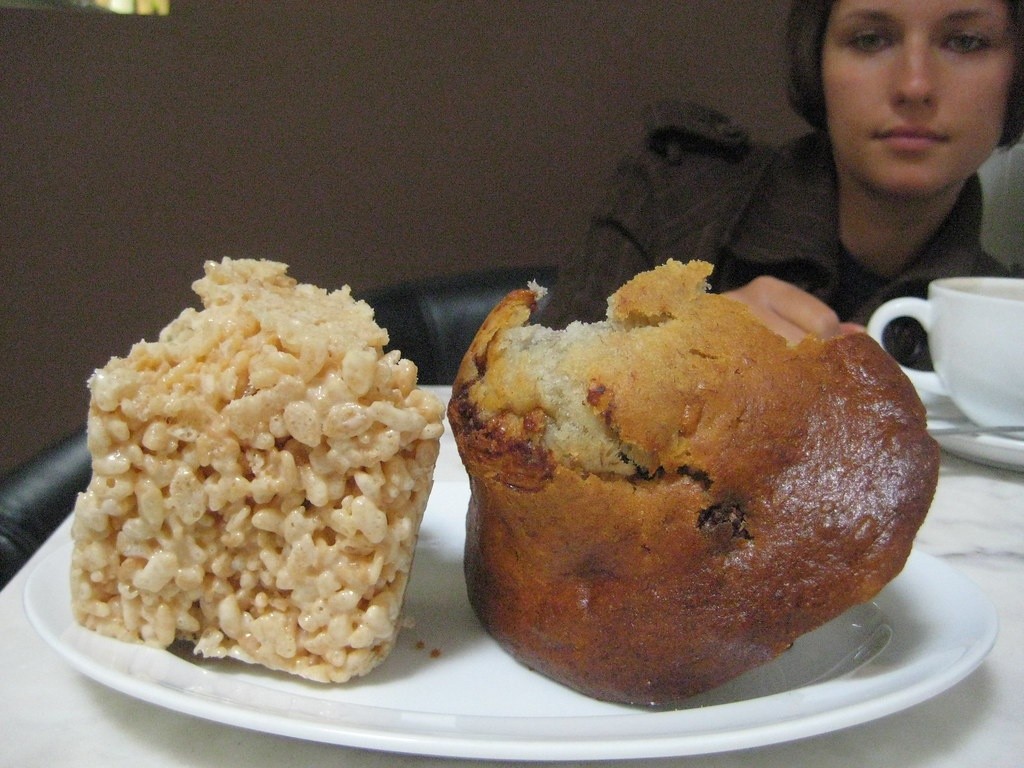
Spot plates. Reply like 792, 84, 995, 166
28, 485, 1000, 759
917, 389, 1024, 475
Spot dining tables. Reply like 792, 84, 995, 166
0, 407, 1024, 768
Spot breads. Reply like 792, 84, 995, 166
447, 259, 942, 708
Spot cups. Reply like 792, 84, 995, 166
867, 277, 1024, 430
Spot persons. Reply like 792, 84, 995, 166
528, 0, 1024, 372
